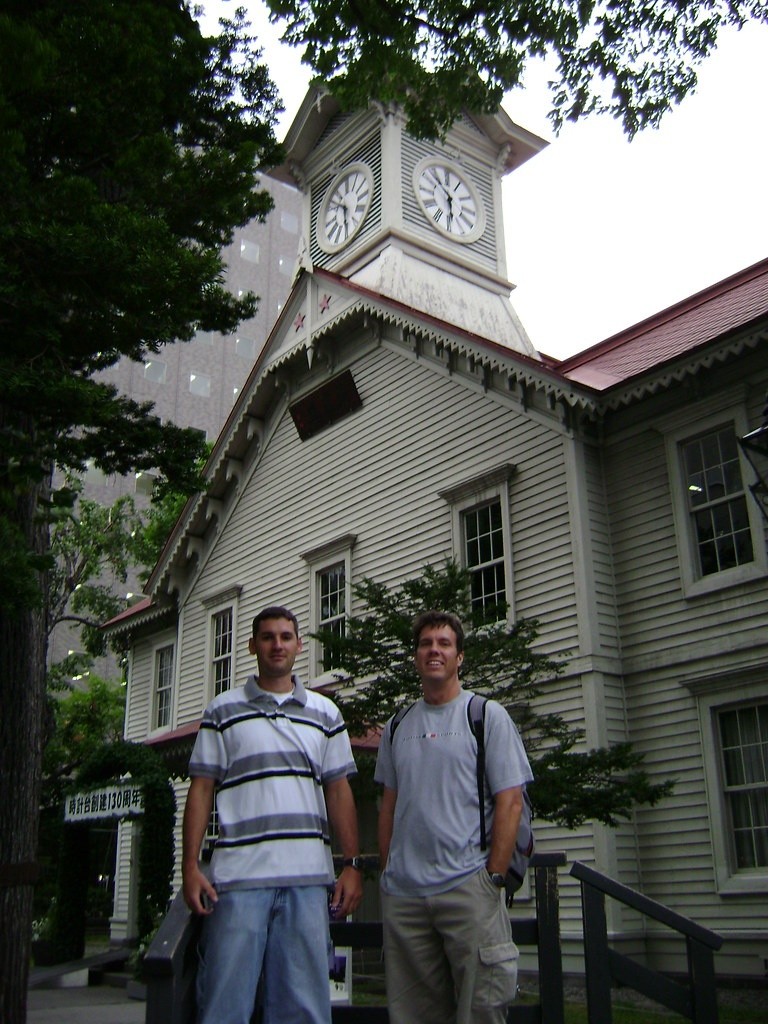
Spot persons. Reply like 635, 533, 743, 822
181, 607, 365, 1024
378, 613, 535, 1023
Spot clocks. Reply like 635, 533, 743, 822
412, 155, 485, 244
315, 161, 374, 255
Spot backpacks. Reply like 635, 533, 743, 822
389, 695, 534, 895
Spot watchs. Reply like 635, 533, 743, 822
488, 870, 505, 888
343, 857, 364, 872
